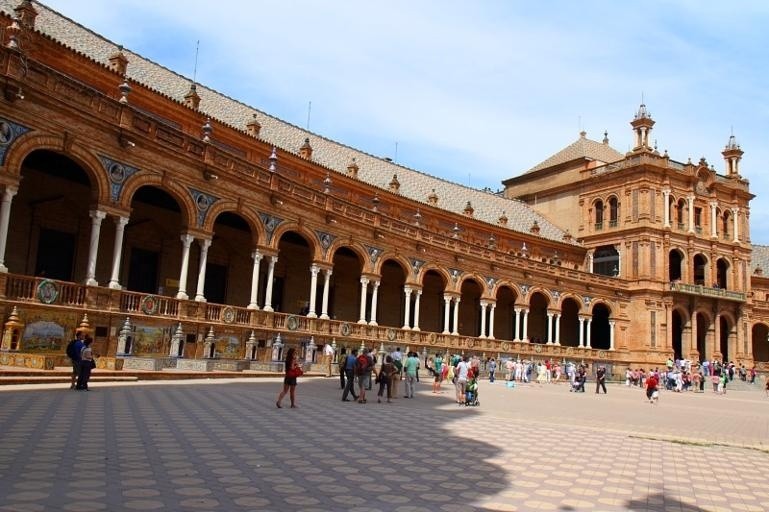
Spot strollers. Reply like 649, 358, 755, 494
570, 376, 585, 392
465, 374, 479, 406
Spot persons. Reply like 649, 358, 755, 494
487, 357, 587, 392
75, 337, 94, 391
625, 358, 756, 404
339, 346, 421, 403
595, 365, 606, 394
426, 353, 480, 404
70, 331, 84, 390
275, 347, 299, 409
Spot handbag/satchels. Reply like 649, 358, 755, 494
466, 380, 478, 391
287, 364, 303, 379
89, 360, 95, 369
67, 339, 78, 358
465, 391, 478, 402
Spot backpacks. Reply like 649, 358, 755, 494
356, 355, 369, 375
